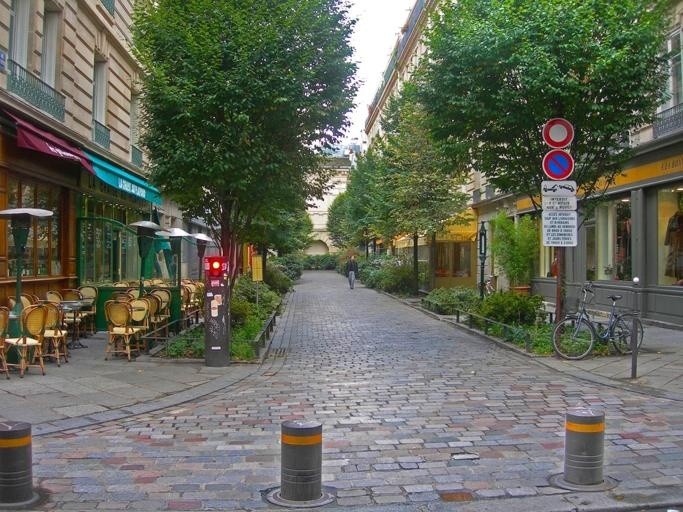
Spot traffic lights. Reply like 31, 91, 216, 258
210, 258, 222, 273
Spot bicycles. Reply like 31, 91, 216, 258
553, 283, 645, 360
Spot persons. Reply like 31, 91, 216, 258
346, 256, 357, 290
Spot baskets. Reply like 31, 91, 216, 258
563, 295, 580, 314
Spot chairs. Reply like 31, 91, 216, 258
0, 279, 205, 379
